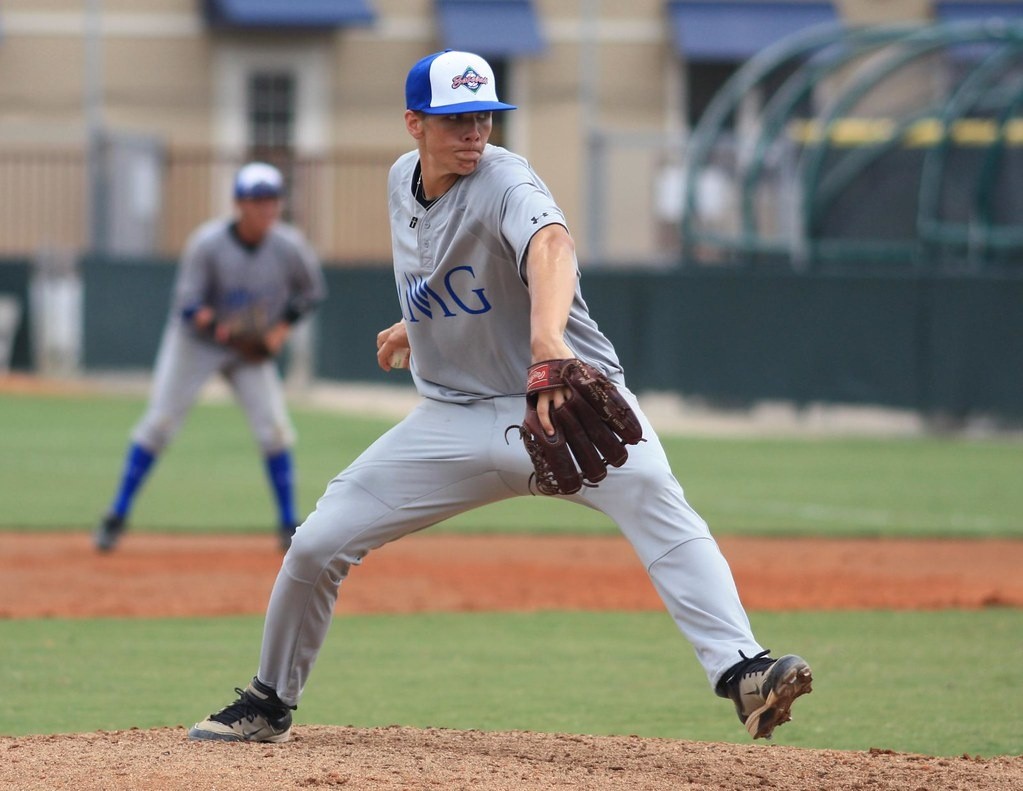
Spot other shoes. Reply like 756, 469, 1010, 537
94, 512, 123, 550
279, 527, 298, 550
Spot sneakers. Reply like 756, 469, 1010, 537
724, 649, 813, 740
187, 675, 297, 743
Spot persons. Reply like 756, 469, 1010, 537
91, 160, 323, 552
180, 48, 816, 744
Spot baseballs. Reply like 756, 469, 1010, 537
389, 348, 411, 371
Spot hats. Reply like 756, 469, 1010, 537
405, 47, 518, 115
234, 161, 287, 199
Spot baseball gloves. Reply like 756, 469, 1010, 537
504, 356, 648, 499
